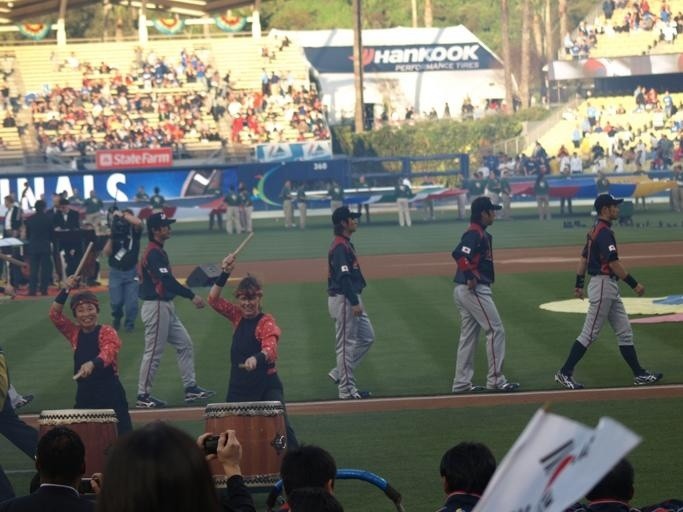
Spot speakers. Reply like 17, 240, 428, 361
185, 263, 223, 288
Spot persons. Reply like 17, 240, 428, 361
205, 171, 437, 236
207, 252, 300, 454
452, 197, 519, 392
432, 441, 499, 512
100, 419, 256, 509
275, 443, 343, 509
0, 35, 333, 157
135, 211, 218, 408
554, 191, 663, 388
564, 1, 682, 61
1, 183, 168, 332
458, 86, 682, 221
327, 206, 376, 399
49, 274, 134, 436
573, 459, 648, 510
0, 333, 106, 511
377, 101, 452, 130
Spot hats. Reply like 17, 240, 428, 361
595, 193, 623, 204
333, 207, 361, 219
147, 213, 176, 226
471, 197, 502, 210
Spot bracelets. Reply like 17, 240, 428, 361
625, 272, 637, 287
254, 351, 267, 368
576, 276, 585, 285
215, 271, 233, 288
53, 288, 72, 303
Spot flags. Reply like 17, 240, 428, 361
473, 408, 645, 511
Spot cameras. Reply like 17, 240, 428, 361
80, 477, 98, 497
201, 434, 229, 456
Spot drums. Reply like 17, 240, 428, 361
204, 400, 286, 488
39, 408, 119, 481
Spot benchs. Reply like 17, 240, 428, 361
563, 0, 683, 59
520, 92, 683, 157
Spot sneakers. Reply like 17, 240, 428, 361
634, 371, 662, 385
499, 383, 520, 391
555, 370, 585, 389
328, 373, 372, 400
10, 396, 33, 411
470, 386, 484, 393
184, 386, 214, 402
113, 319, 135, 332
136, 395, 168, 409
